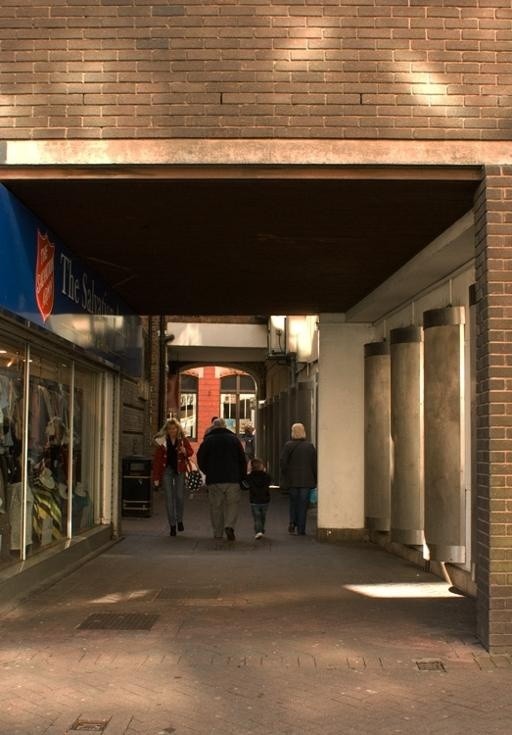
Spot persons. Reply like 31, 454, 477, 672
239, 425, 255, 462
196, 418, 248, 542
204, 416, 219, 434
150, 417, 195, 537
241, 456, 274, 541
278, 422, 318, 536
31, 467, 64, 547
56, 481, 79, 538
72, 481, 92, 534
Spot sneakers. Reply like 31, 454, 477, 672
254, 531, 263, 540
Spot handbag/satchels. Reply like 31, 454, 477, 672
309, 487, 317, 504
183, 456, 206, 493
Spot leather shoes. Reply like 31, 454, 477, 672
224, 527, 236, 541
288, 522, 307, 535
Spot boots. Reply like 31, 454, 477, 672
177, 521, 184, 532
170, 525, 176, 536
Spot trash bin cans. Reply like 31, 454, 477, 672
121, 455, 154, 517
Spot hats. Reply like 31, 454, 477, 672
290, 422, 307, 439
